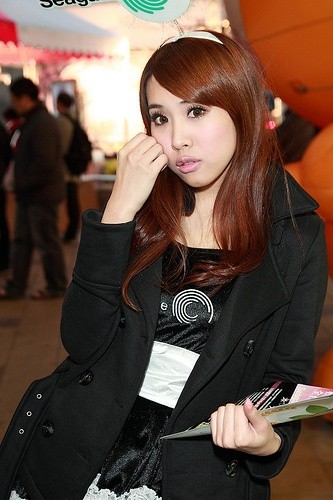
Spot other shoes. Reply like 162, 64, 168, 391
0, 287, 24, 299
31, 287, 65, 300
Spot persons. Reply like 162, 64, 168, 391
0, 28, 333, 500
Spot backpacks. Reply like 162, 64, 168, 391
59, 112, 92, 178
